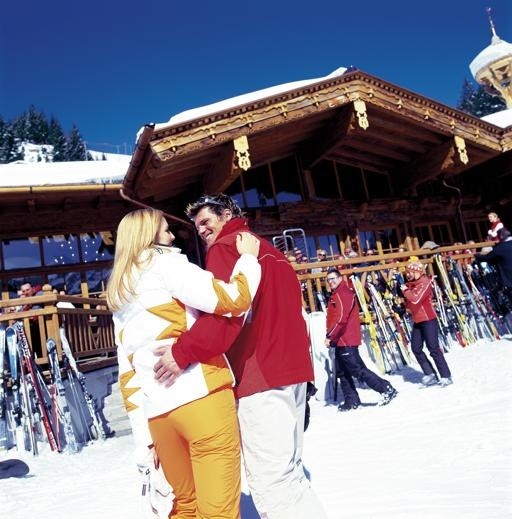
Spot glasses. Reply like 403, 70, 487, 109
197, 195, 222, 206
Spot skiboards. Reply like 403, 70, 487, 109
1, 321, 107, 456
350, 254, 512, 376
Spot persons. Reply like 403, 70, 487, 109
280, 211, 512, 409
103, 208, 263, 519
150, 192, 314, 519
0, 280, 102, 362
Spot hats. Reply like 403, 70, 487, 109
405, 262, 423, 272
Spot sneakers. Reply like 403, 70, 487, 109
379, 389, 398, 406
338, 403, 358, 412
424, 374, 453, 387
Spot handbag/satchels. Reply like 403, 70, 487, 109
135, 454, 176, 519
